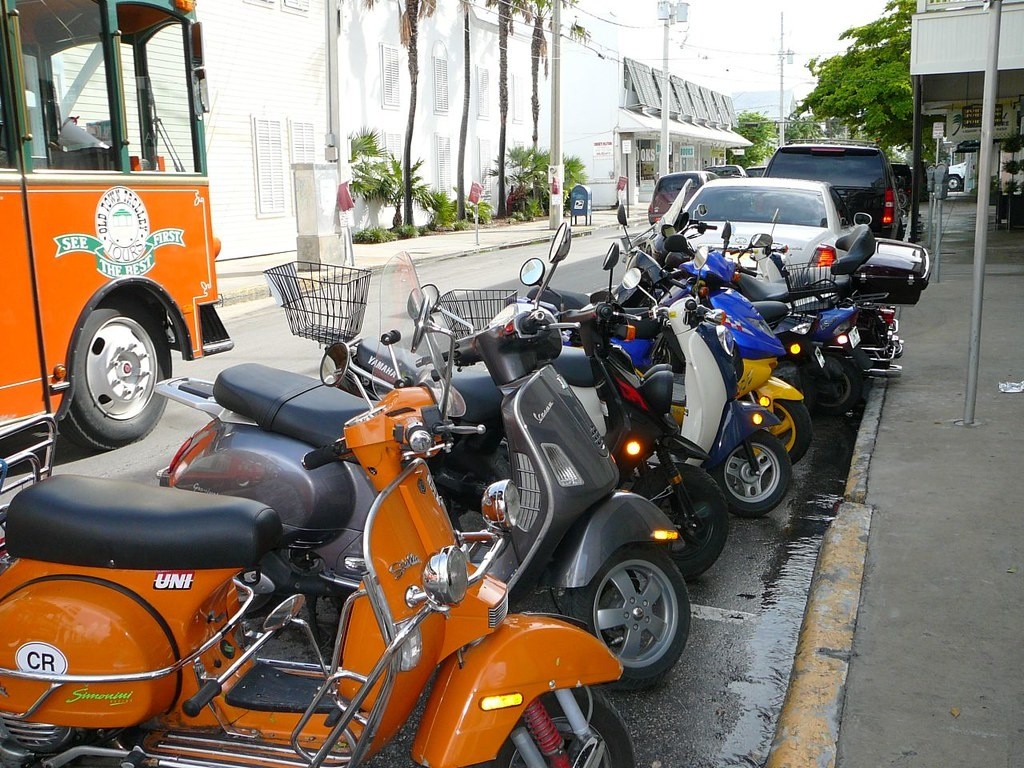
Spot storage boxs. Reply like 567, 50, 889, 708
852, 237, 931, 304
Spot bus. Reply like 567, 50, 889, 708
0, 0, 236, 458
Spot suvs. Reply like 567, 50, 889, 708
889, 161, 913, 209
754, 137, 909, 243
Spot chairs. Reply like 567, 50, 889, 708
794, 224, 875, 298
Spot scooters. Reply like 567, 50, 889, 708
146, 173, 931, 694
1, 248, 641, 768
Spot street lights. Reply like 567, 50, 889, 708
655, 0, 690, 180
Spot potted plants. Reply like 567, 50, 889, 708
995, 134, 1024, 231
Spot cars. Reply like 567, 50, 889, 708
947, 161, 967, 191
702, 164, 749, 183
677, 175, 854, 289
647, 170, 722, 228
745, 165, 768, 178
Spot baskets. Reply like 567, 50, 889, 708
439, 288, 519, 340
262, 260, 372, 345
781, 260, 839, 313
